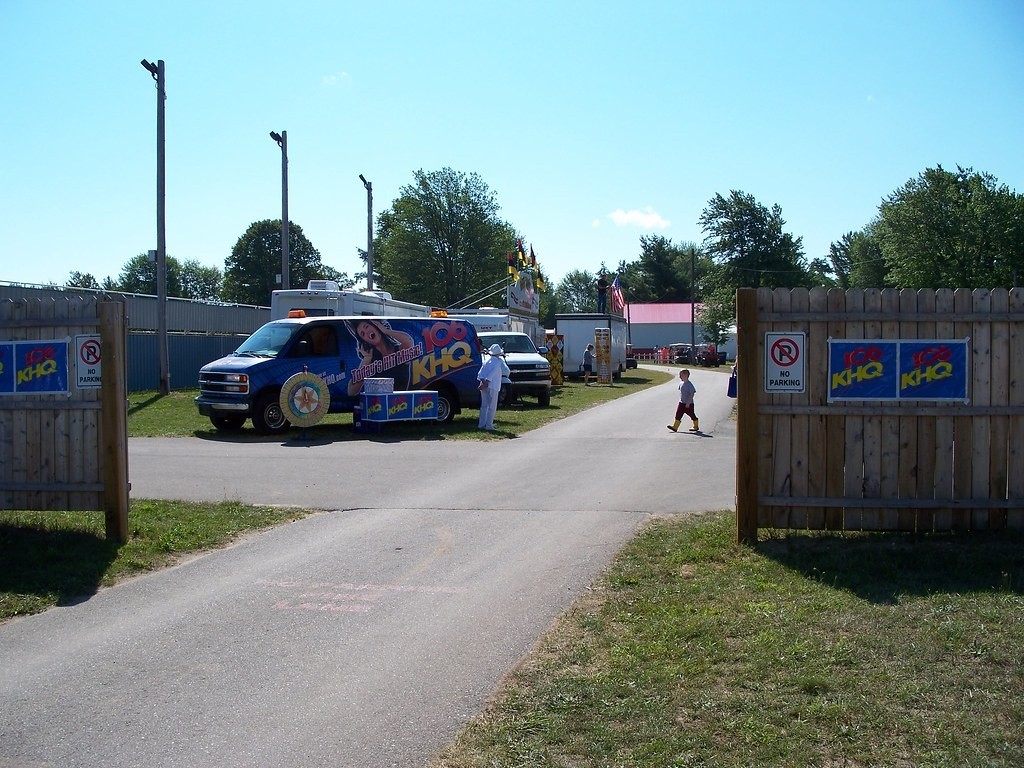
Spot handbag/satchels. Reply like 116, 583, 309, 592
727, 366, 737, 398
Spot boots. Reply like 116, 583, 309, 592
689, 419, 699, 431
667, 418, 681, 432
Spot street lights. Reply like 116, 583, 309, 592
270, 128, 290, 290
358, 175, 374, 294
139, 58, 168, 391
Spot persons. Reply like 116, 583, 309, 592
477, 344, 505, 430
667, 370, 699, 432
597, 274, 610, 313
348, 320, 414, 395
654, 344, 699, 366
583, 344, 597, 385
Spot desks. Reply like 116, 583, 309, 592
360, 391, 439, 435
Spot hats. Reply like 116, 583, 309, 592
488, 343, 504, 355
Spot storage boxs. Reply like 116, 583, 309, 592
363, 378, 395, 393
353, 406, 386, 434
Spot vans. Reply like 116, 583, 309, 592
193, 309, 488, 438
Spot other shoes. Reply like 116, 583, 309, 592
485, 424, 496, 430
478, 424, 485, 429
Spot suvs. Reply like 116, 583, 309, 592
474, 330, 554, 409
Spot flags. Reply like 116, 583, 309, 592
518, 240, 528, 266
611, 277, 625, 312
529, 247, 538, 269
508, 254, 520, 281
537, 271, 546, 292
